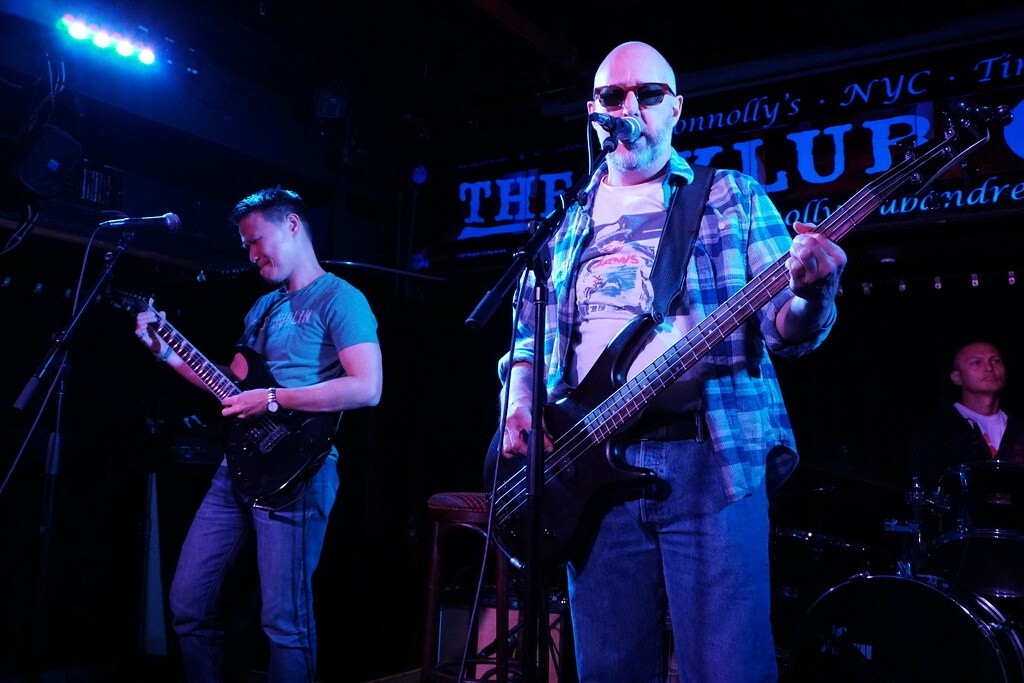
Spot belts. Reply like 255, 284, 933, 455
628, 410, 710, 441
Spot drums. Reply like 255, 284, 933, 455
766, 525, 877, 662
786, 570, 1023, 683
930, 458, 1024, 600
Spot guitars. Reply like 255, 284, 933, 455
106, 288, 335, 511
477, 99, 1016, 599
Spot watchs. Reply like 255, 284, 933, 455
267, 388, 279, 413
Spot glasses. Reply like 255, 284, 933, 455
593, 83, 676, 107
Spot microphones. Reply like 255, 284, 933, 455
478, 634, 516, 660
98, 212, 182, 235
590, 112, 643, 145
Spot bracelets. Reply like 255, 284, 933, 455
162, 347, 172, 362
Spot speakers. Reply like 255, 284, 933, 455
473, 597, 563, 683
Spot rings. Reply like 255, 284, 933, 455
140, 333, 144, 338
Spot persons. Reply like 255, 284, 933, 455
498, 42, 848, 683
476, 430, 561, 683
895, 341, 1024, 527
133, 187, 383, 683
